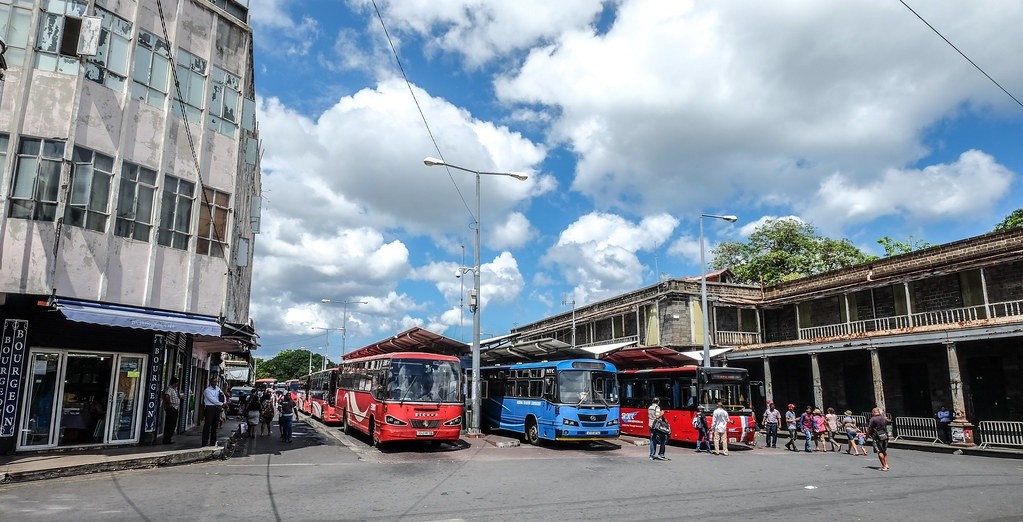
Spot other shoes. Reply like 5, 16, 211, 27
268, 432, 272, 435
650, 457, 654, 461
707, 450, 713, 454
658, 456, 668, 461
696, 449, 701, 452
283, 437, 292, 442
879, 466, 889, 472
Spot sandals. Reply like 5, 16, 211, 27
164, 440, 175, 444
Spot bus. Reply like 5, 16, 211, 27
337, 351, 466, 448
255, 379, 277, 392
286, 368, 342, 424
617, 365, 765, 446
463, 358, 620, 445
277, 382, 285, 392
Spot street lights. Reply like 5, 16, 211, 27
312, 327, 345, 369
322, 299, 368, 352
700, 214, 738, 368
424, 157, 529, 435
302, 347, 322, 371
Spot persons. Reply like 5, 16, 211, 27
826, 407, 841, 452
237, 389, 299, 442
202, 378, 227, 448
866, 408, 890, 471
937, 406, 952, 444
812, 409, 827, 452
785, 404, 801, 452
840, 410, 859, 456
711, 402, 730, 455
800, 406, 814, 452
162, 378, 183, 445
647, 398, 670, 461
695, 405, 713, 453
856, 429, 867, 455
761, 403, 781, 448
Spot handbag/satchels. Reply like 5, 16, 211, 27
878, 432, 888, 440
263, 412, 274, 421
218, 390, 224, 402
836, 415, 842, 429
652, 416, 670, 435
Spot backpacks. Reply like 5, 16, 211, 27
692, 414, 703, 429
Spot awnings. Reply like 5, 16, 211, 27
57, 304, 221, 337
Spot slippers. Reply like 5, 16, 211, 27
766, 443, 868, 455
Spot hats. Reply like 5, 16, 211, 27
697, 404, 705, 409
844, 410, 852, 417
813, 409, 821, 414
788, 404, 795, 408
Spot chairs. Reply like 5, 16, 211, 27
504, 384, 539, 398
620, 396, 672, 409
388, 381, 438, 402
30, 414, 67, 446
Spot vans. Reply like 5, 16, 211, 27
229, 387, 254, 411
